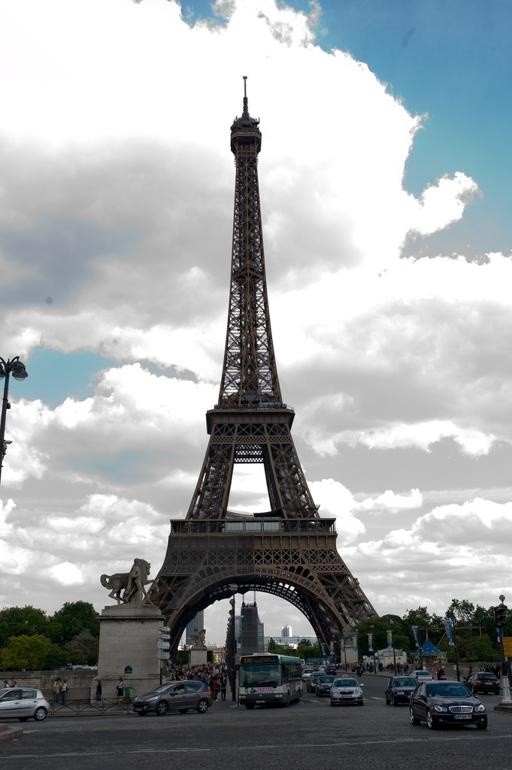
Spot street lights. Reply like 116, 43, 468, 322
0, 355, 29, 477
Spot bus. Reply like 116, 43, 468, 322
235, 653, 305, 711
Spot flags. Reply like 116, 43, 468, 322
339, 619, 456, 655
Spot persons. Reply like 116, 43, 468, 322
11, 680, 19, 687
94, 677, 105, 703
50, 677, 70, 705
3, 680, 10, 687
116, 675, 126, 705
160, 661, 236, 706
335, 659, 512, 691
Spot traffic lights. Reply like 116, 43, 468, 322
494, 606, 507, 627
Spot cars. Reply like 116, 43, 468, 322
0, 687, 53, 721
301, 663, 337, 697
408, 679, 488, 732
327, 677, 365, 706
385, 675, 419, 706
410, 670, 434, 687
464, 671, 501, 696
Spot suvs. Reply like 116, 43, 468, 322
133, 679, 213, 716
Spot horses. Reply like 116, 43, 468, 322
99, 558, 160, 606
